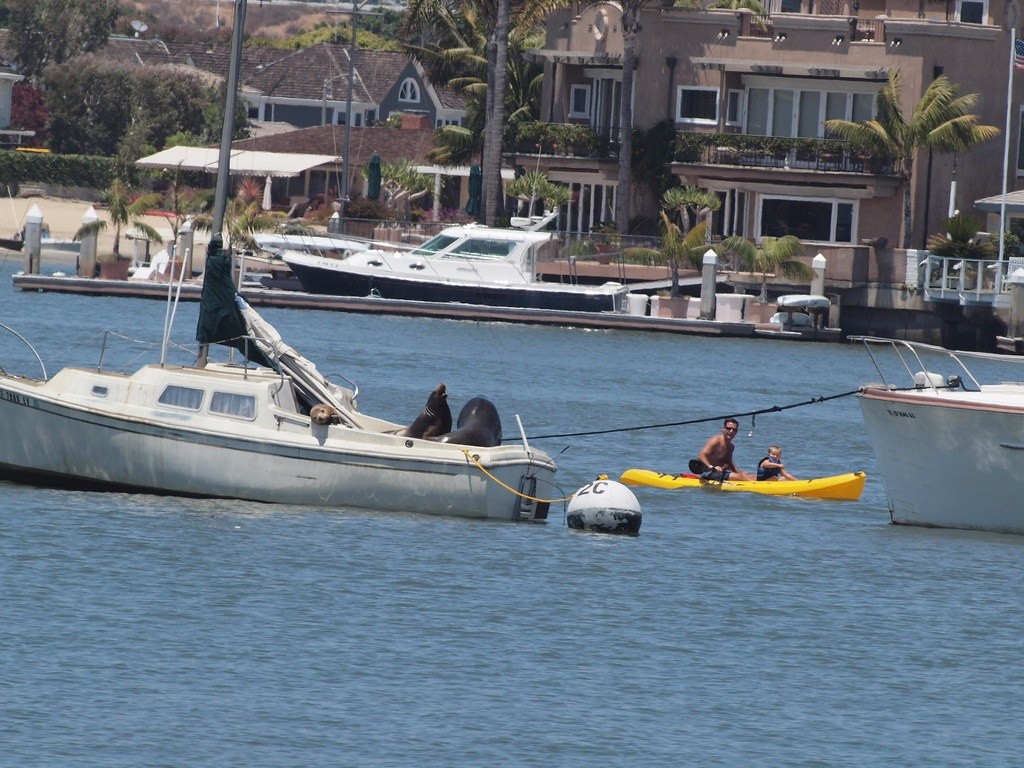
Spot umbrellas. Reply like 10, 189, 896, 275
466, 164, 482, 216
366, 155, 382, 200
261, 174, 273, 212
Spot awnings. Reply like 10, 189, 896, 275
133, 145, 343, 178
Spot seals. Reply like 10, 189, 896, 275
381, 383, 503, 447
310, 403, 347, 426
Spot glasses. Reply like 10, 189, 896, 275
725, 427, 737, 432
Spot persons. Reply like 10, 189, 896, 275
697, 419, 798, 481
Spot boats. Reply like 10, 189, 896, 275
251, 142, 630, 315
622, 469, 866, 501
845, 335, 1024, 536
0, 0, 558, 525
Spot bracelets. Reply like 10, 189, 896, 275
709, 465, 714, 469
